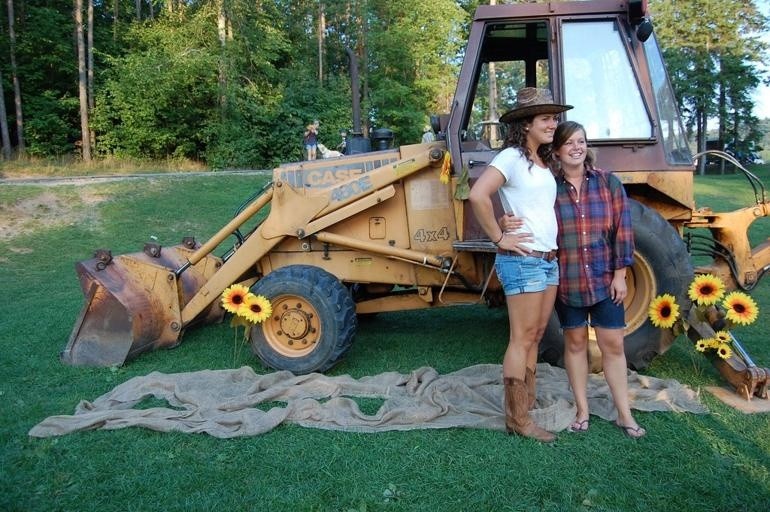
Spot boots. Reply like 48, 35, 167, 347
504, 366, 558, 442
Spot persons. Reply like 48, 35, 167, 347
303, 120, 319, 161
467, 86, 574, 443
498, 120, 646, 439
421, 125, 434, 144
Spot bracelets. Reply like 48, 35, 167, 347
493, 231, 504, 244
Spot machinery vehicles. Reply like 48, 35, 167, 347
64, 0, 770, 420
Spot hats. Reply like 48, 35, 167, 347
499, 87, 572, 123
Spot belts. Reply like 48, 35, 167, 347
497, 247, 556, 262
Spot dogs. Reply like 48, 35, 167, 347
316, 143, 344, 159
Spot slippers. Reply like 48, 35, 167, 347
571, 417, 589, 432
616, 418, 645, 439
